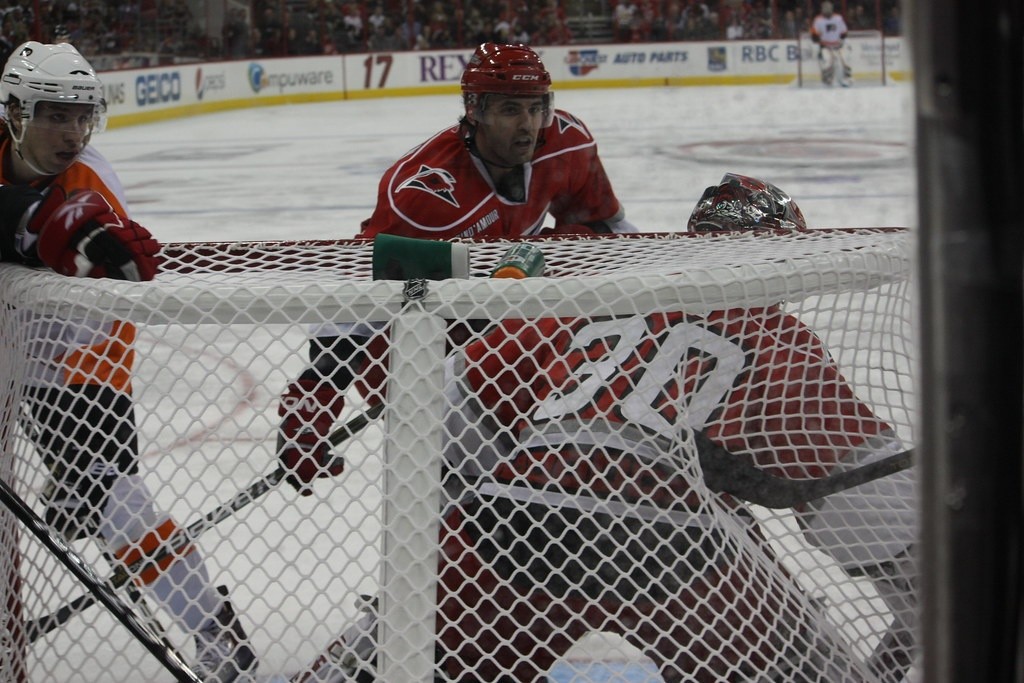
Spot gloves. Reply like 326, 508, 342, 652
26, 184, 161, 281
278, 380, 344, 496
40, 464, 114, 539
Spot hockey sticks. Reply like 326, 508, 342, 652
668, 427, 918, 510
0, 384, 388, 683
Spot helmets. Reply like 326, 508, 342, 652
0, 40, 107, 126
688, 173, 806, 232
461, 43, 552, 127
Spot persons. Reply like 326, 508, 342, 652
0, 40, 258, 683
0, 0, 253, 80
272, 36, 924, 683
606, 0, 901, 42
252, 0, 575, 56
811, 1, 855, 86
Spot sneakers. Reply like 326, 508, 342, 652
189, 585, 258, 683
